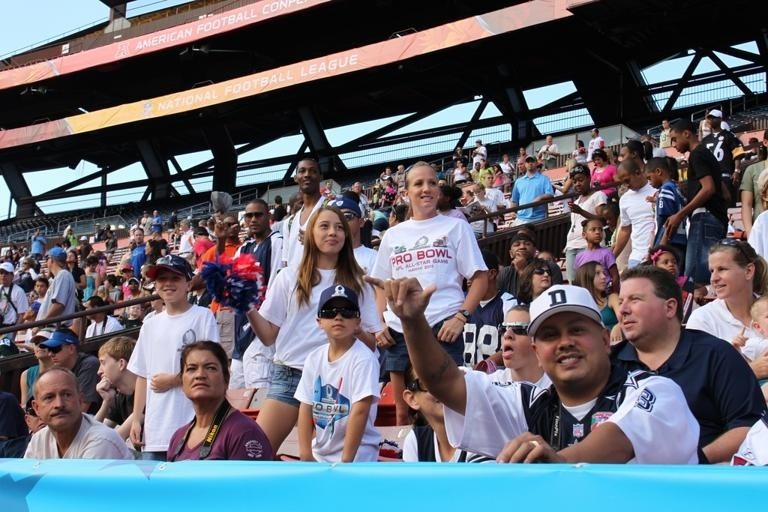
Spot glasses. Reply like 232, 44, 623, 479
407, 378, 430, 392
318, 307, 360, 318
535, 269, 552, 276
27, 409, 38, 417
245, 212, 264, 218
47, 345, 62, 353
344, 212, 360, 221
119, 269, 130, 273
527, 160, 535, 163
498, 324, 529, 335
713, 239, 751, 262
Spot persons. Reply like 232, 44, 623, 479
362, 273, 700, 465
1, 110, 768, 466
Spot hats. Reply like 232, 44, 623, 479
705, 109, 722, 118
128, 277, 139, 283
0, 336, 19, 355
43, 247, 66, 258
39, 330, 80, 349
318, 285, 359, 311
26, 395, 34, 410
331, 197, 362, 215
525, 155, 537, 162
80, 236, 87, 240
527, 284, 605, 337
0, 262, 14, 273
146, 255, 192, 281
30, 327, 55, 342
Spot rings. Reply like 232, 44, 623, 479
385, 277, 395, 281
529, 440, 539, 447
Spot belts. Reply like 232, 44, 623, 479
691, 207, 710, 217
